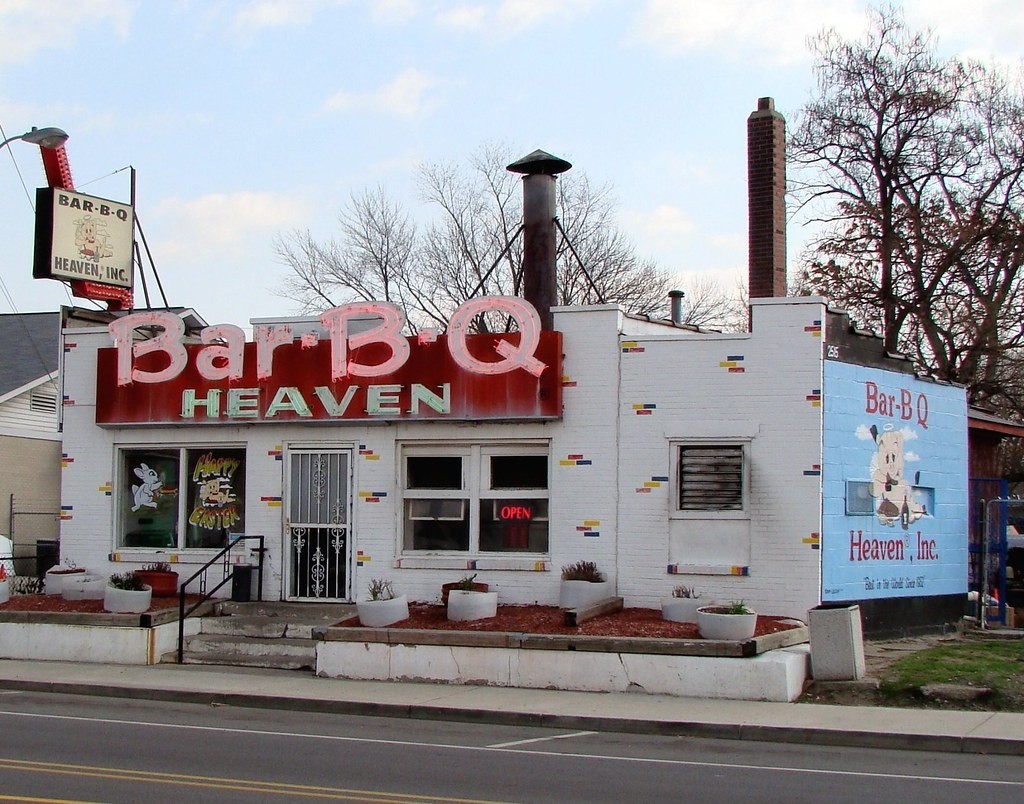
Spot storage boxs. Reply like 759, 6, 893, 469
986, 605, 1015, 627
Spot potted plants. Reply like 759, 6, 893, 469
103, 572, 152, 614
660, 586, 705, 624
695, 599, 757, 641
559, 560, 609, 609
135, 560, 180, 596
46, 560, 107, 601
442, 574, 498, 622
355, 578, 409, 627
